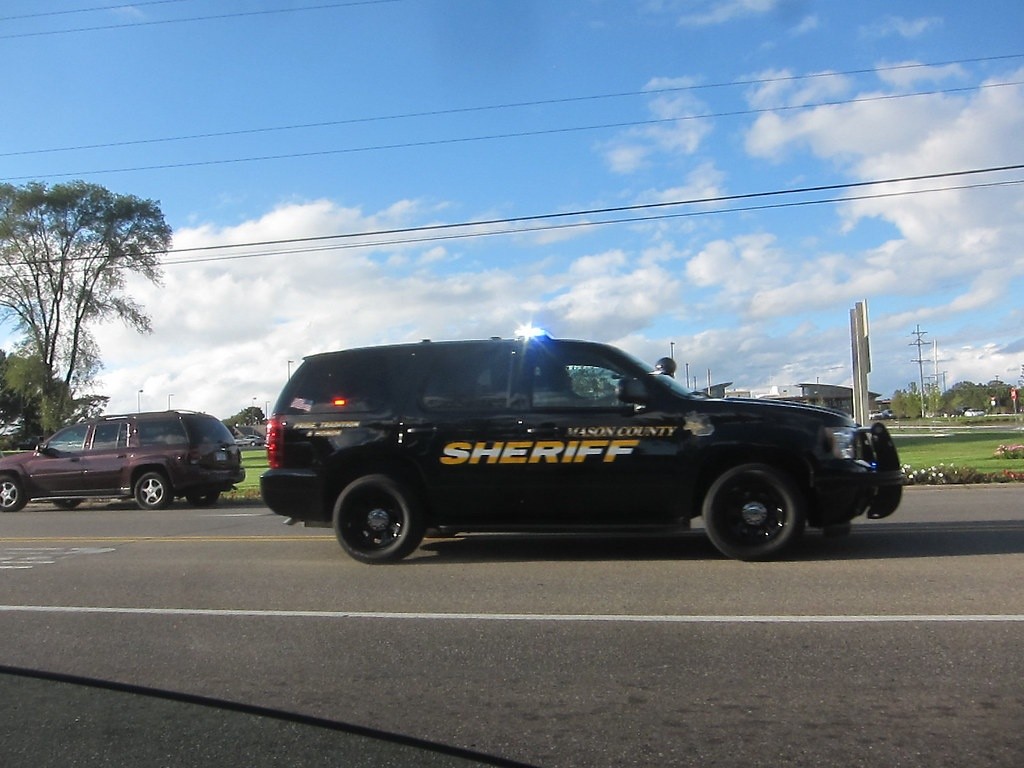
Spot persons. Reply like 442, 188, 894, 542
542, 361, 620, 407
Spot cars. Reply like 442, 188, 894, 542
882, 410, 896, 417
234, 434, 263, 447
866, 408, 884, 420
964, 409, 985, 418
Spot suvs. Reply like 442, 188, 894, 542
0, 406, 247, 512
257, 336, 907, 564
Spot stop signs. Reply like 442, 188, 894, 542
1009, 388, 1017, 399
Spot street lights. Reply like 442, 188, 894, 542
685, 363, 691, 390
265, 401, 270, 417
287, 360, 294, 381
251, 397, 258, 426
167, 393, 175, 410
137, 389, 143, 413
670, 341, 677, 362
